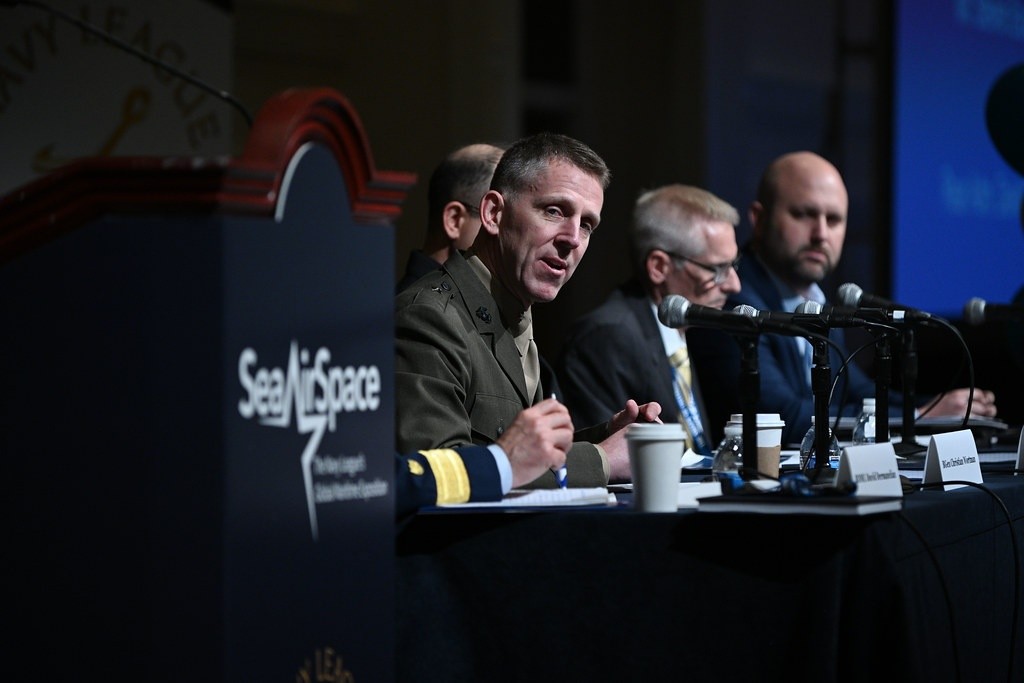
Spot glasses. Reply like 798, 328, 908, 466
663, 251, 740, 284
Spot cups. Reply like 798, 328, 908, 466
623, 423, 687, 514
729, 414, 786, 483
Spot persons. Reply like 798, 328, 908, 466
394, 134, 662, 488
555, 183, 742, 454
396, 144, 507, 294
727, 150, 998, 441
393, 399, 573, 518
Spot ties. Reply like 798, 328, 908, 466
803, 340, 812, 384
670, 346, 693, 451
522, 323, 540, 408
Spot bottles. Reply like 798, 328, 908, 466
851, 398, 891, 446
711, 427, 745, 483
801, 416, 841, 477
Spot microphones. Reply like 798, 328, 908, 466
657, 282, 931, 339
964, 297, 1024, 327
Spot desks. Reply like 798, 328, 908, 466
398, 434, 1024, 683
